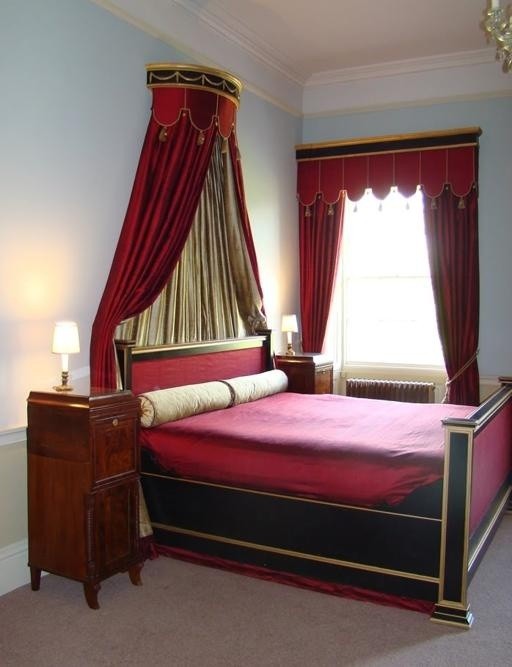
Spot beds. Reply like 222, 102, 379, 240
116, 330, 512, 629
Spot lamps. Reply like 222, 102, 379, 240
282, 314, 298, 355
52, 321, 80, 391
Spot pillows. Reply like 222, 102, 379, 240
224, 368, 289, 407
137, 380, 233, 429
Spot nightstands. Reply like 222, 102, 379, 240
276, 353, 334, 394
27, 387, 143, 609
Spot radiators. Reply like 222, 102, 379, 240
345, 378, 436, 403
